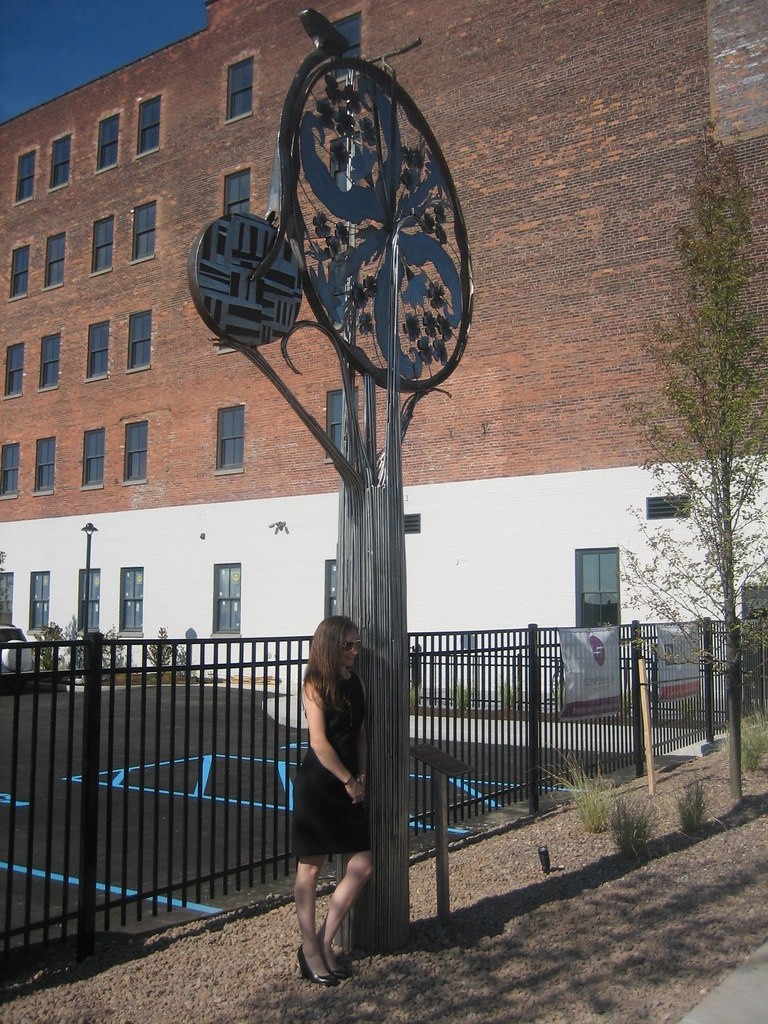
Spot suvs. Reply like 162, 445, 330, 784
0, 623, 36, 693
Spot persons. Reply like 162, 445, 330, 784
286, 615, 377, 989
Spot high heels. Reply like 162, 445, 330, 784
297, 946, 337, 985
330, 969, 348, 978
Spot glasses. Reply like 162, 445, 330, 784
342, 640, 361, 650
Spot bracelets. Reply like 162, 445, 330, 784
344, 774, 353, 785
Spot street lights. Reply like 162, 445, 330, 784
81, 523, 98, 637
